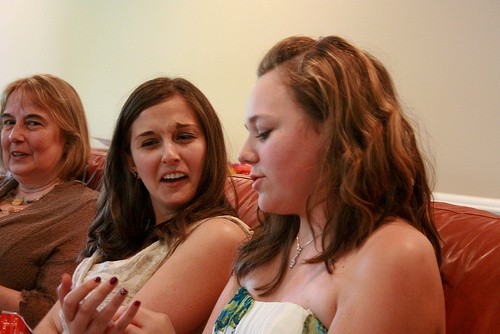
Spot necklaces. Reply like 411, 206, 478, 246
290, 233, 327, 268
11, 193, 36, 207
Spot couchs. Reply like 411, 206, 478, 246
80, 149, 500, 334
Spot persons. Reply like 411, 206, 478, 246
0, 74, 100, 331
31, 78, 256, 334
200, 35, 446, 334
